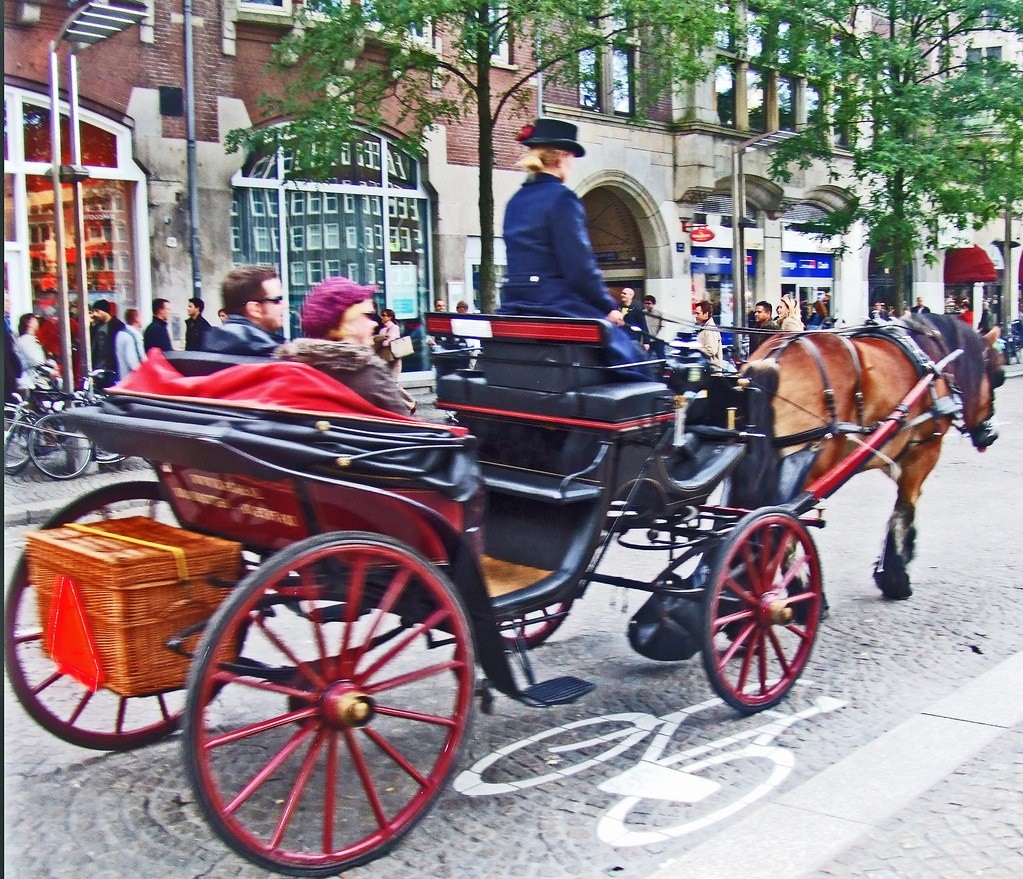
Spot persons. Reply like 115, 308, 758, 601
4, 289, 229, 420
492, 116, 688, 396
276, 276, 481, 568
204, 267, 288, 358
374, 299, 481, 380
620, 291, 999, 377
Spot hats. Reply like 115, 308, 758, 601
92, 300, 110, 313
519, 119, 585, 157
302, 277, 374, 337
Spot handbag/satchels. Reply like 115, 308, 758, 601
377, 347, 395, 362
389, 336, 415, 358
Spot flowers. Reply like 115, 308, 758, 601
514, 122, 534, 142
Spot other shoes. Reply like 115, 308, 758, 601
410, 402, 417, 416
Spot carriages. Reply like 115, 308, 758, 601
7, 308, 1006, 879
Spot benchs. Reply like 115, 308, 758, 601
478, 438, 617, 503
101, 345, 471, 552
417, 311, 673, 418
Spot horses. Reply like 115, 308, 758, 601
721, 311, 1006, 654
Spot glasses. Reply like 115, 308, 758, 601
256, 296, 282, 304
381, 314, 387, 316
362, 313, 374, 320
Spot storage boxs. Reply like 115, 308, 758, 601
26, 514, 243, 698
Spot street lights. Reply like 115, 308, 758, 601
730, 129, 800, 362
46, 1, 152, 476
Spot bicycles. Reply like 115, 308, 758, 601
3, 363, 129, 481
